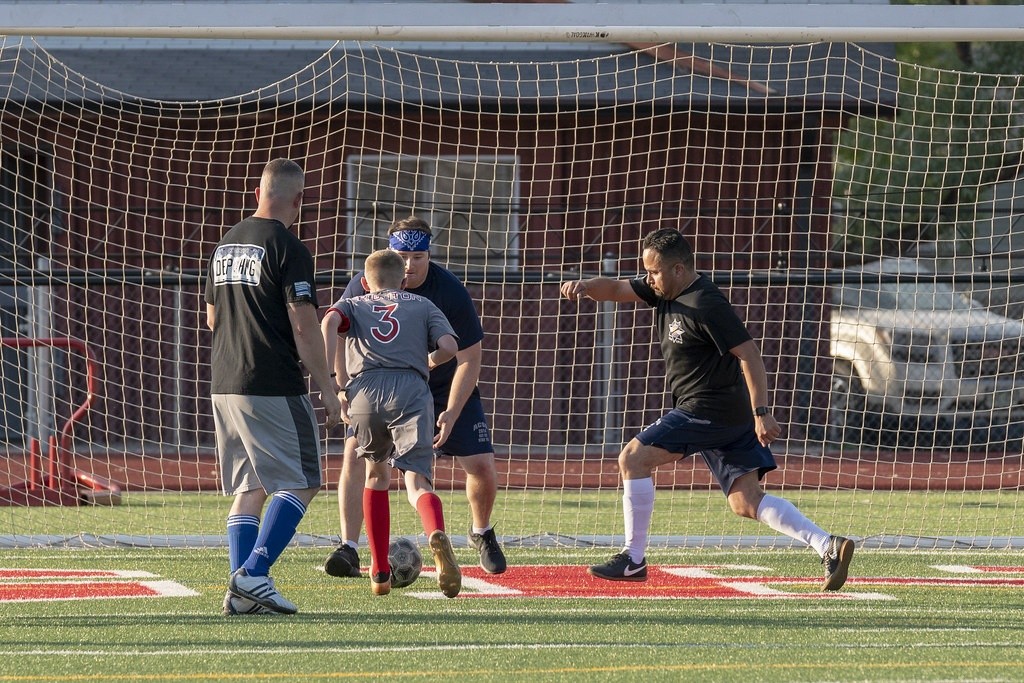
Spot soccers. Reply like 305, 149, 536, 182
386, 537, 422, 589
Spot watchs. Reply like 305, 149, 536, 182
753, 406, 770, 416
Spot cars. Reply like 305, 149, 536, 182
828, 258, 1024, 451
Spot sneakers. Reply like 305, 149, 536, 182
587, 549, 647, 581
467, 521, 506, 574
228, 566, 296, 614
821, 535, 855, 591
223, 590, 280, 615
428, 530, 462, 598
325, 534, 360, 577
369, 559, 396, 595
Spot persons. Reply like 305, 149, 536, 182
560, 227, 856, 591
321, 251, 462, 598
204, 158, 341, 615
324, 216, 506, 577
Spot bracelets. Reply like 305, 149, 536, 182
340, 389, 345, 391
330, 373, 336, 377
